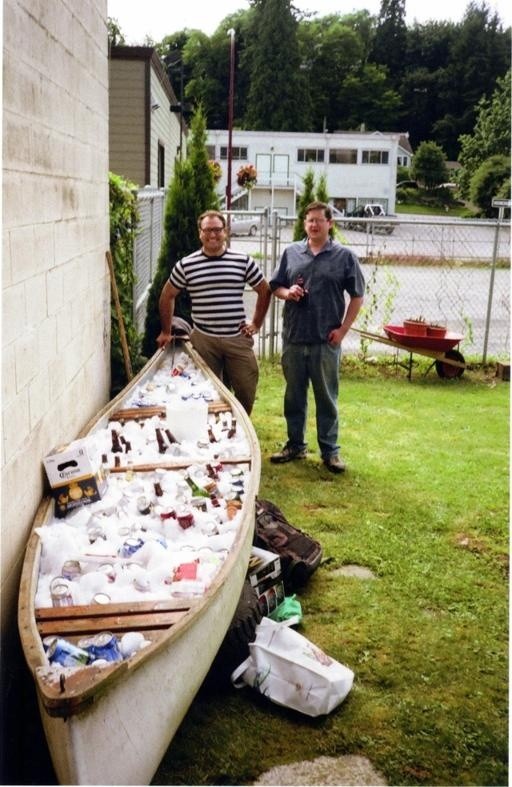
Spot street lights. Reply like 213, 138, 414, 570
226, 28, 235, 248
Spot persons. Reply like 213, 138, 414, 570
269, 201, 366, 473
155, 210, 271, 417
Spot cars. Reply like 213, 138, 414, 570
230, 215, 262, 237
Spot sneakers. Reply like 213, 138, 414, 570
324, 455, 345, 472
270, 444, 307, 463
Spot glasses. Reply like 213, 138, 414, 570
200, 226, 225, 233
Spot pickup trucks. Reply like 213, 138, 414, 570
344, 203, 401, 234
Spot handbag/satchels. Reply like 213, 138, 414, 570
231, 616, 355, 717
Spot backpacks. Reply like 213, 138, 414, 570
253, 496, 323, 580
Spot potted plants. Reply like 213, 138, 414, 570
403, 314, 447, 338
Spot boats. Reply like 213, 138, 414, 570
18, 316, 261, 785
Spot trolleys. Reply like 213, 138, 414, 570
348, 324, 467, 381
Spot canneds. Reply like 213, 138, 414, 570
137, 497, 153, 514
224, 469, 244, 509
63, 560, 81, 580
123, 538, 144, 558
170, 364, 183, 377
43, 631, 123, 669
208, 461, 221, 474
160, 507, 194, 528
203, 482, 223, 500
192, 497, 206, 511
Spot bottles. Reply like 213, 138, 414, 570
295, 270, 312, 307
101, 408, 238, 515
